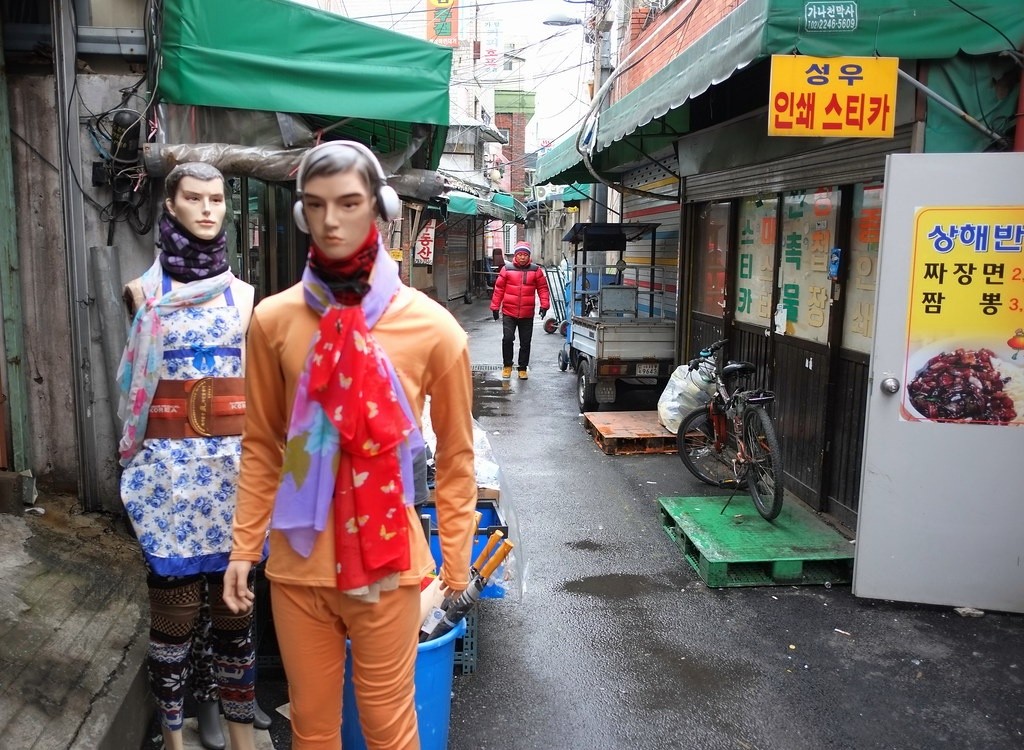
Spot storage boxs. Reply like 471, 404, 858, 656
418, 501, 508, 601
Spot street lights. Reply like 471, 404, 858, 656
539, 12, 599, 222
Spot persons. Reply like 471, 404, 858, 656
124, 138, 478, 749
490, 241, 550, 379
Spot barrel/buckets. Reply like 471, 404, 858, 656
340, 616, 468, 750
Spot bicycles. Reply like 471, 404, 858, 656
676, 338, 785, 521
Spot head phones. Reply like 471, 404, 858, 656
292, 141, 401, 235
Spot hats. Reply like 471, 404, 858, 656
514, 241, 533, 254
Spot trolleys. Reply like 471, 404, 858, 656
535, 251, 570, 336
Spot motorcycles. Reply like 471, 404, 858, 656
560, 221, 677, 413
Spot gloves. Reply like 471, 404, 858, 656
538, 307, 548, 320
493, 310, 499, 320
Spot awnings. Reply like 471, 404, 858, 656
148, 0, 452, 172
445, 189, 528, 226
521, 185, 590, 210
534, 0, 1024, 197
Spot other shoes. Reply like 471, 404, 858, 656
518, 371, 528, 378
501, 367, 512, 378
